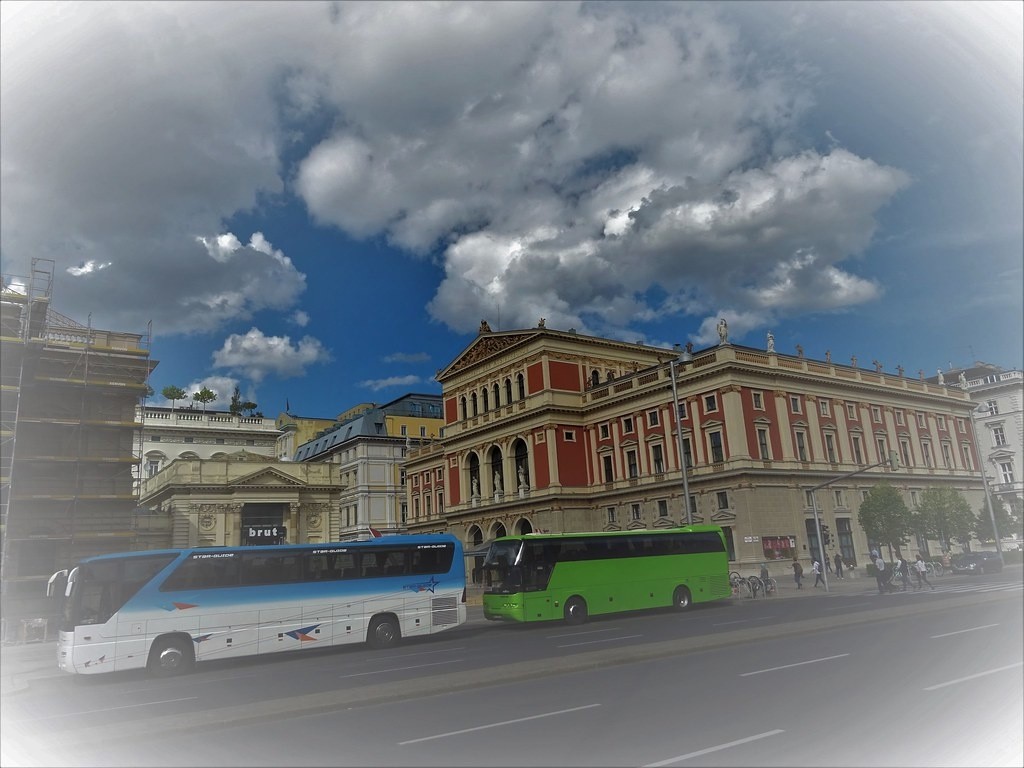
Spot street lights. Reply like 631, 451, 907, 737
671, 350, 695, 526
969, 402, 1007, 568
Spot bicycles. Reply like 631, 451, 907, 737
728, 571, 780, 600
885, 552, 986, 588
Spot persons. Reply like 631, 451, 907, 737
810, 558, 825, 587
835, 554, 844, 580
868, 545, 935, 595
787, 556, 805, 590
760, 565, 768, 586
307, 559, 328, 580
825, 552, 832, 574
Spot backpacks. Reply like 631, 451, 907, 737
815, 562, 822, 571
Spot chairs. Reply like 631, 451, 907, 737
337, 563, 404, 580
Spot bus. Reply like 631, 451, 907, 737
471, 523, 734, 626
46, 534, 467, 681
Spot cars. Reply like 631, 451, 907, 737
952, 550, 1002, 574
